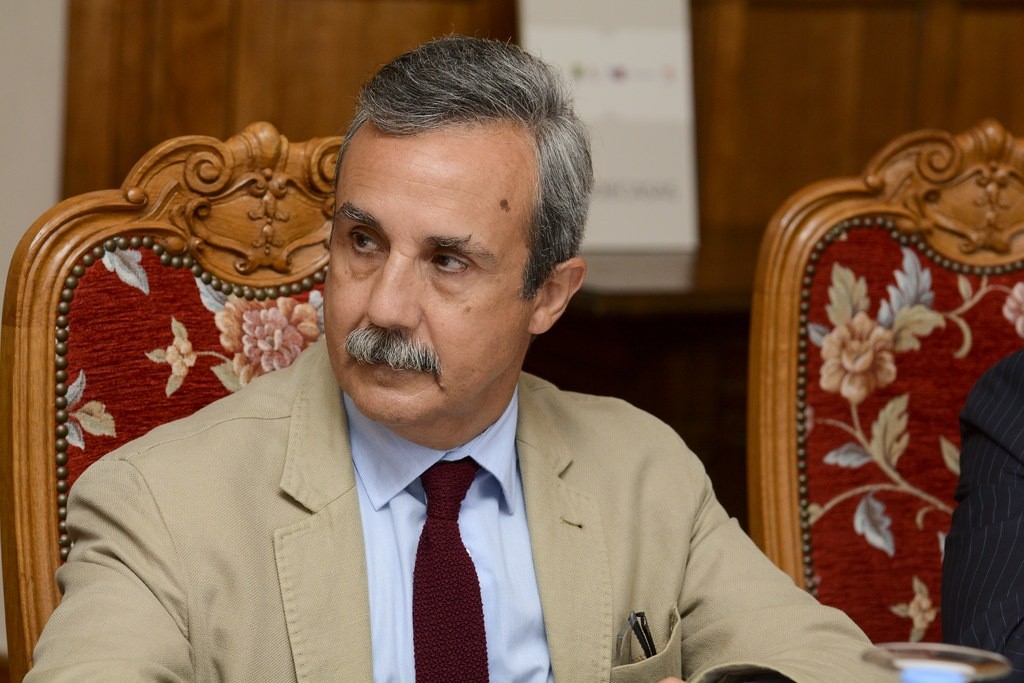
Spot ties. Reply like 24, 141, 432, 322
412, 456, 490, 683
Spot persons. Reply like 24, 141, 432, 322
940, 348, 1024, 672
23, 37, 902, 683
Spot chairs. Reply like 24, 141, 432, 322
0, 122, 352, 682
745, 119, 1024, 644
